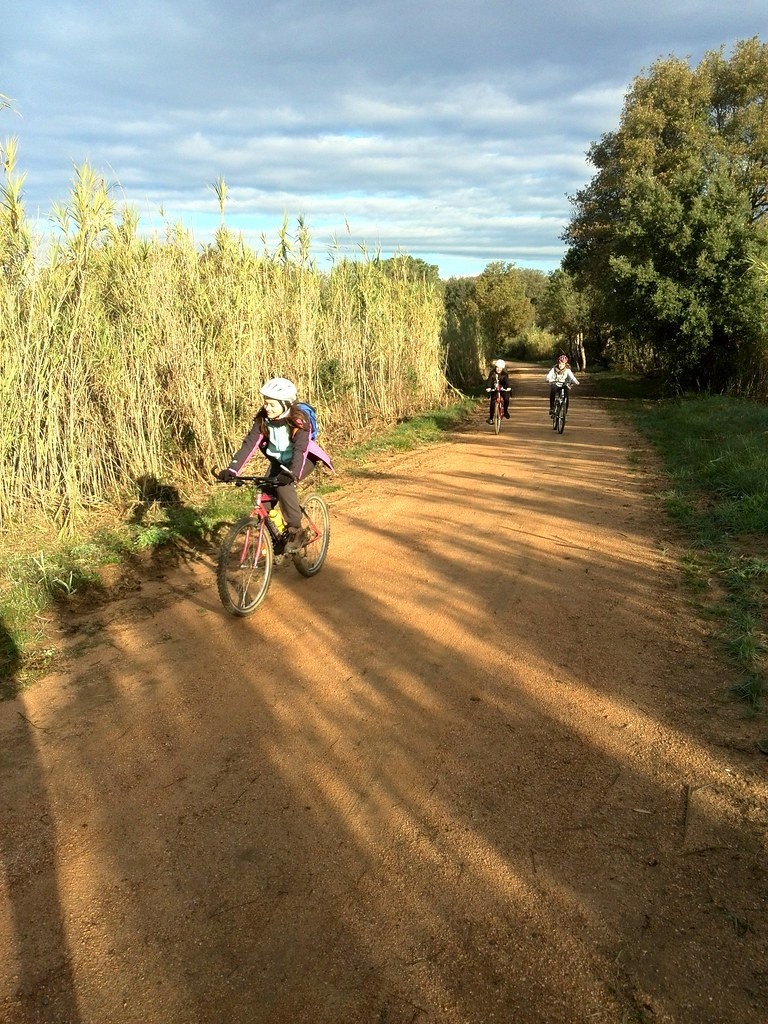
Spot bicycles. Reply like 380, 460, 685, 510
211, 464, 331, 617
548, 380, 575, 434
490, 383, 511, 434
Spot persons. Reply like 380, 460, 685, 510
546, 355, 580, 423
217, 378, 335, 552
486, 359, 511, 424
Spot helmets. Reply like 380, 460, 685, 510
558, 356, 569, 364
495, 359, 506, 368
260, 378, 297, 401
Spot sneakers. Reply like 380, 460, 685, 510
284, 526, 306, 553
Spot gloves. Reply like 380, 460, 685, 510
271, 470, 292, 486
507, 388, 511, 391
219, 470, 236, 483
485, 387, 491, 392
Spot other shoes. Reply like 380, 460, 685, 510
565, 416, 567, 422
504, 411, 510, 419
486, 418, 492, 424
549, 407, 555, 415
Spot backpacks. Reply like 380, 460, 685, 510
261, 403, 315, 442
